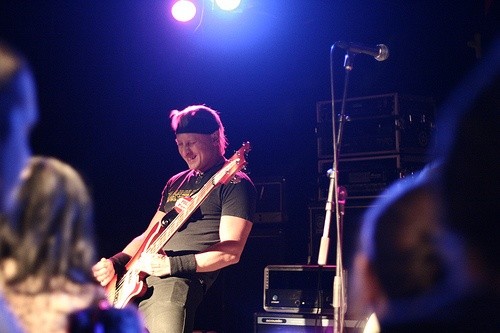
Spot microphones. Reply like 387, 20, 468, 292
336, 41, 389, 62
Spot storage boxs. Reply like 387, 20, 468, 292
318, 154, 434, 203
308, 206, 372, 265
316, 93, 437, 159
253, 313, 362, 333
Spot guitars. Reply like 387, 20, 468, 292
113, 141, 253, 310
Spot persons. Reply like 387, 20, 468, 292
0, 42, 499, 333
91, 104, 257, 333
